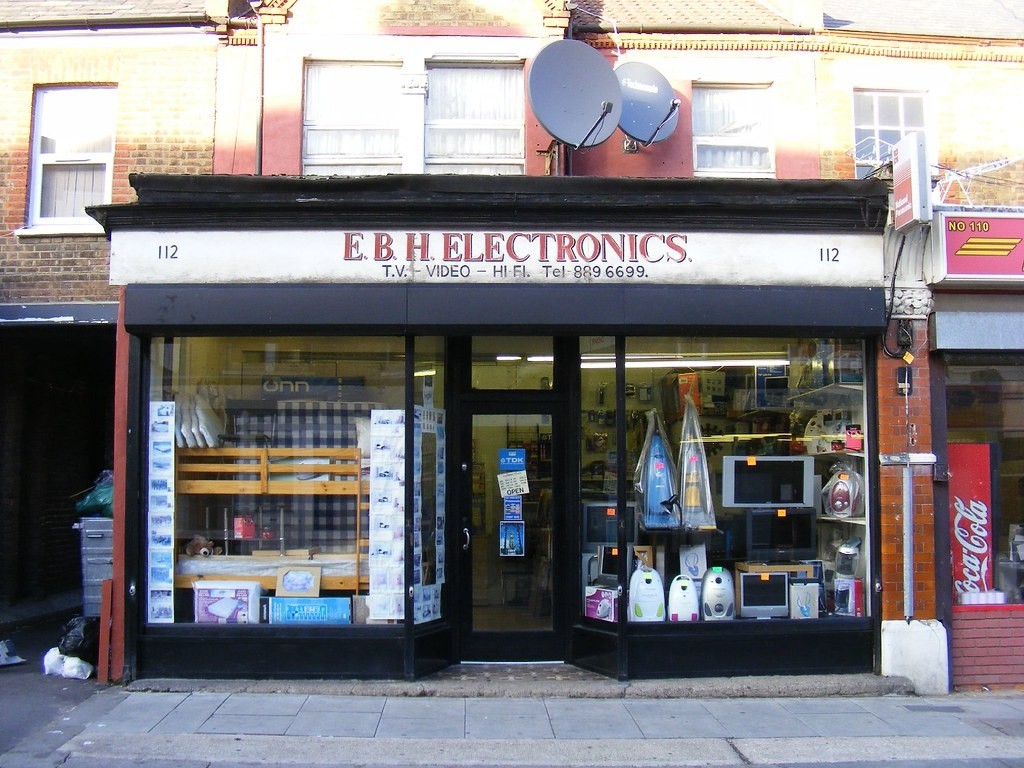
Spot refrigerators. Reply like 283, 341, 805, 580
946, 442, 997, 604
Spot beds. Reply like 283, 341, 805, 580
175, 446, 370, 495
173, 552, 370, 587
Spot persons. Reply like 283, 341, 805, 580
505, 448, 541, 607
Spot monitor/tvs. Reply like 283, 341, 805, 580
581, 500, 638, 553
598, 545, 633, 587
744, 509, 816, 564
723, 456, 814, 508
740, 572, 788, 619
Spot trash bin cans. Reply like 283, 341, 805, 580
71, 517, 113, 617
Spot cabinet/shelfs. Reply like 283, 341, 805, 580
788, 338, 872, 616
501, 420, 641, 606
204, 503, 285, 556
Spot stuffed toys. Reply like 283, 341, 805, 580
186, 537, 223, 557
